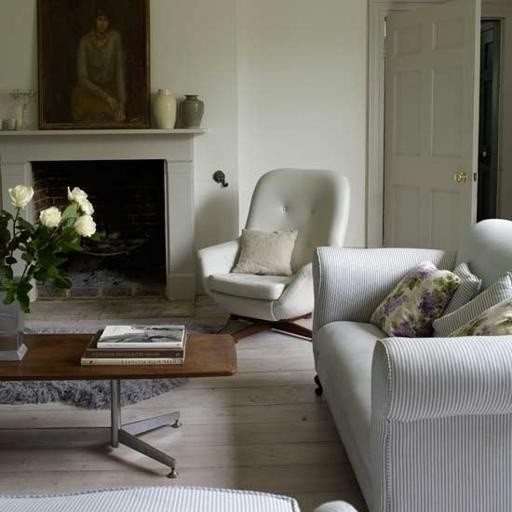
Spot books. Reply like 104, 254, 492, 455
81, 325, 187, 365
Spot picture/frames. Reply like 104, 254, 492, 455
34, 0, 152, 131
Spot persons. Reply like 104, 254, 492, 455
71, 8, 127, 121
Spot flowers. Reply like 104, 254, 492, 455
0, 185, 102, 313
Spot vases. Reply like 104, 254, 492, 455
0, 290, 29, 361
153, 89, 177, 130
180, 94, 204, 129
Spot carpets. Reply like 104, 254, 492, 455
0, 320, 194, 410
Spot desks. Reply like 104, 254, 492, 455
0, 333, 238, 479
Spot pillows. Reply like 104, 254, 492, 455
369, 262, 460, 337
446, 297, 512, 338
432, 271, 512, 337
231, 228, 299, 276
439, 262, 483, 318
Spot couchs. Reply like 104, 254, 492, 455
193, 167, 351, 342
0, 484, 300, 512
310, 218, 512, 512
